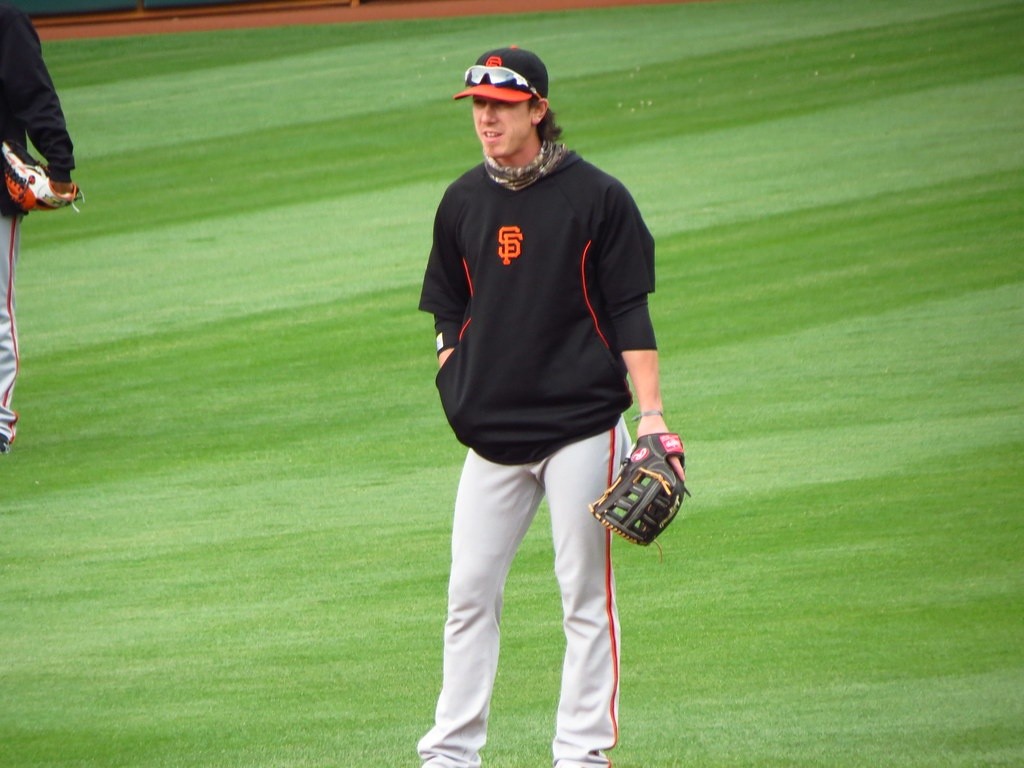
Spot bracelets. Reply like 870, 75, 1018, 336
632, 410, 663, 422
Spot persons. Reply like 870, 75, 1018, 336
0, 0, 76, 454
418, 47, 686, 768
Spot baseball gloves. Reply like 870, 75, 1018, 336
1, 140, 80, 213
588, 435, 688, 548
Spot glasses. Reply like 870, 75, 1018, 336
463, 65, 543, 101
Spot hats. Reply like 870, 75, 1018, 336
452, 45, 550, 104
